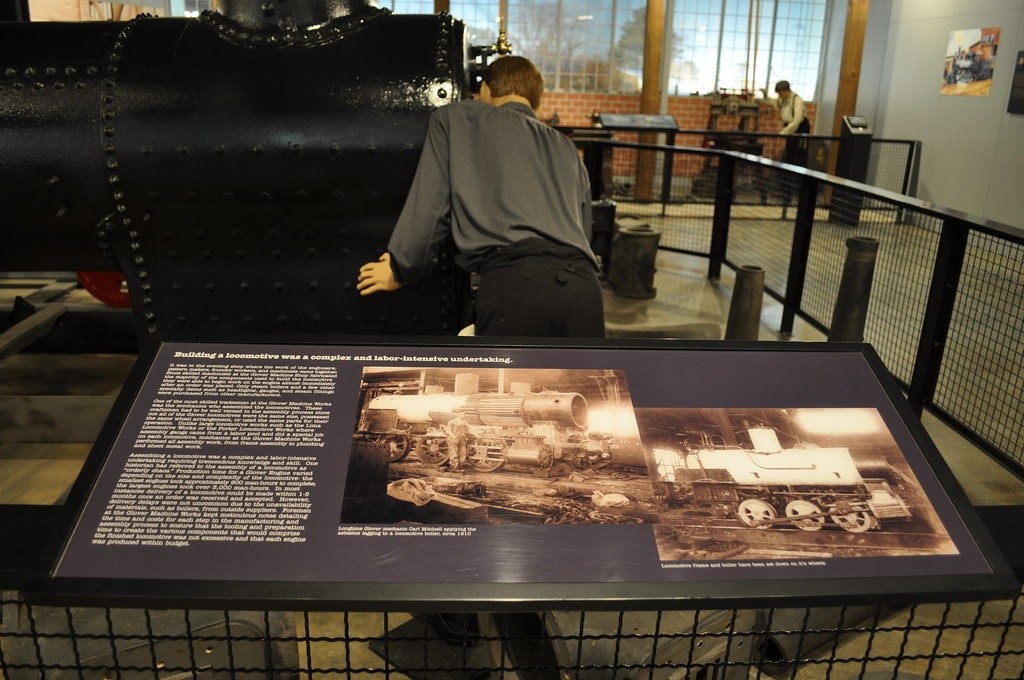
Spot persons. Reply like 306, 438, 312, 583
356, 55, 607, 648
764, 80, 812, 207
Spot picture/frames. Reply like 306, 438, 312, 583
20, 326, 1015, 609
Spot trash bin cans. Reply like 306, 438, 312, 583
610, 225, 662, 301
